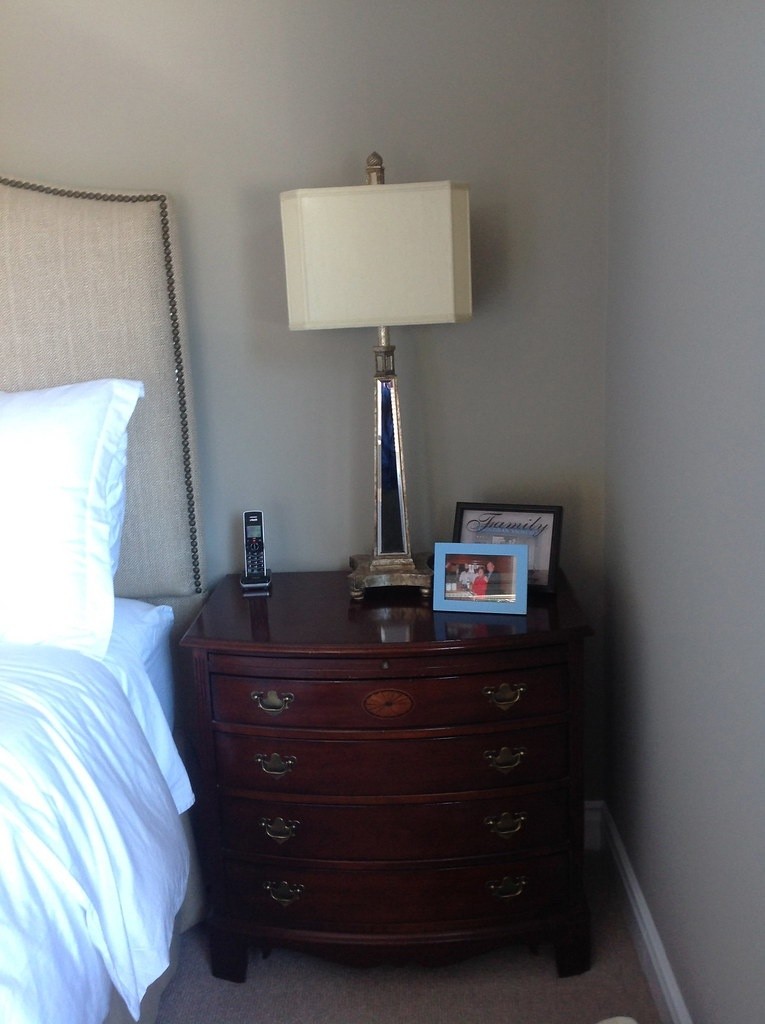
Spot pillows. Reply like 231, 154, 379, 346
0, 379, 147, 663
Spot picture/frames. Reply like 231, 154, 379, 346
452, 501, 564, 595
432, 542, 527, 615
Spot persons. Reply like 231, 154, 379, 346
459, 561, 500, 595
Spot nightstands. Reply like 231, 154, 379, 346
178, 570, 593, 979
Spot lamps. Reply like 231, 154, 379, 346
278, 148, 474, 604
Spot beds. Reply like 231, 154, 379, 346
2, 176, 217, 1024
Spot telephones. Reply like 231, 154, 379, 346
238, 509, 273, 590
